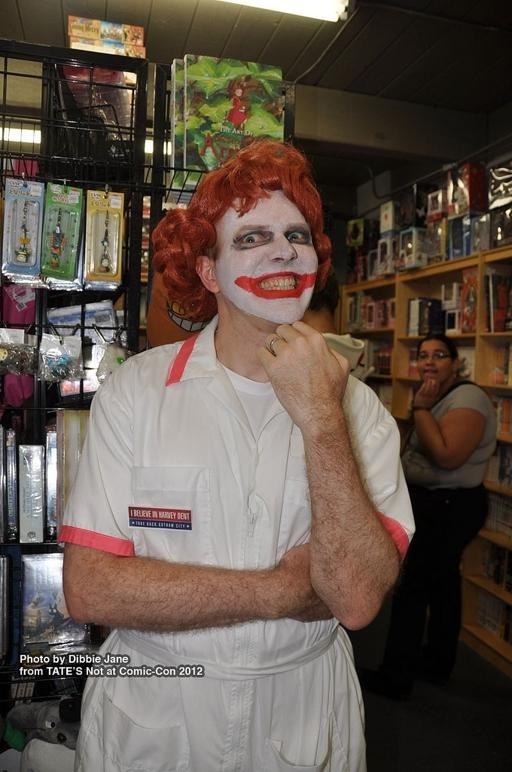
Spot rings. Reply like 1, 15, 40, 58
269, 337, 284, 353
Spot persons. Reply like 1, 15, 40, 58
376, 335, 496, 700
55, 139, 417, 769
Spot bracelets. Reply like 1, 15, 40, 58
412, 406, 426, 411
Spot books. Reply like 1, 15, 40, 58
0, 409, 89, 666
170, 54, 284, 203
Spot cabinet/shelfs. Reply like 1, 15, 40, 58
328, 235, 511, 684
